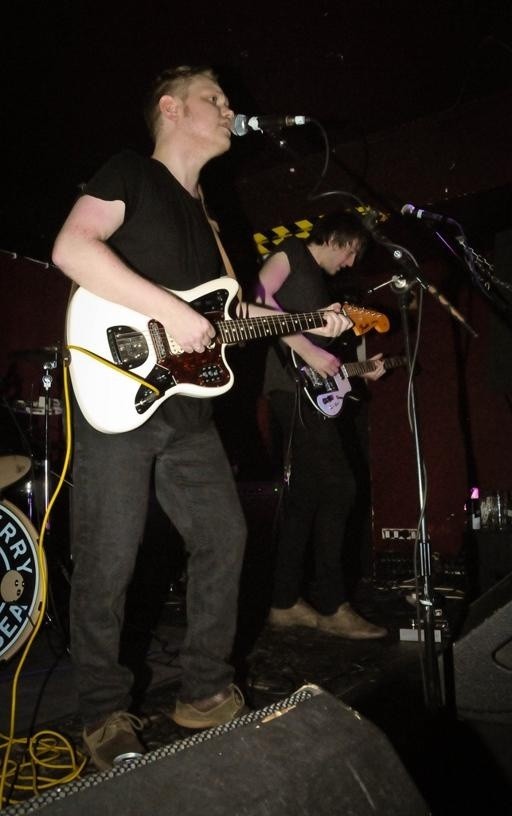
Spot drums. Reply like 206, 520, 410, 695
1, 455, 41, 536
9, 397, 65, 480
0, 498, 48, 663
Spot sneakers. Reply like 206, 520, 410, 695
171, 680, 282, 730
315, 601, 390, 642
267, 595, 323, 631
79, 706, 152, 779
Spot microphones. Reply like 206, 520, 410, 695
401, 203, 456, 225
393, 250, 420, 282
227, 114, 305, 137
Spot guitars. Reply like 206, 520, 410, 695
67, 275, 390, 433
287, 344, 409, 419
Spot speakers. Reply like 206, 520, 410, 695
432, 565, 512, 724
0, 681, 437, 816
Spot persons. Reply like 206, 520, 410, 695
53, 63, 355, 775
246, 207, 389, 640
